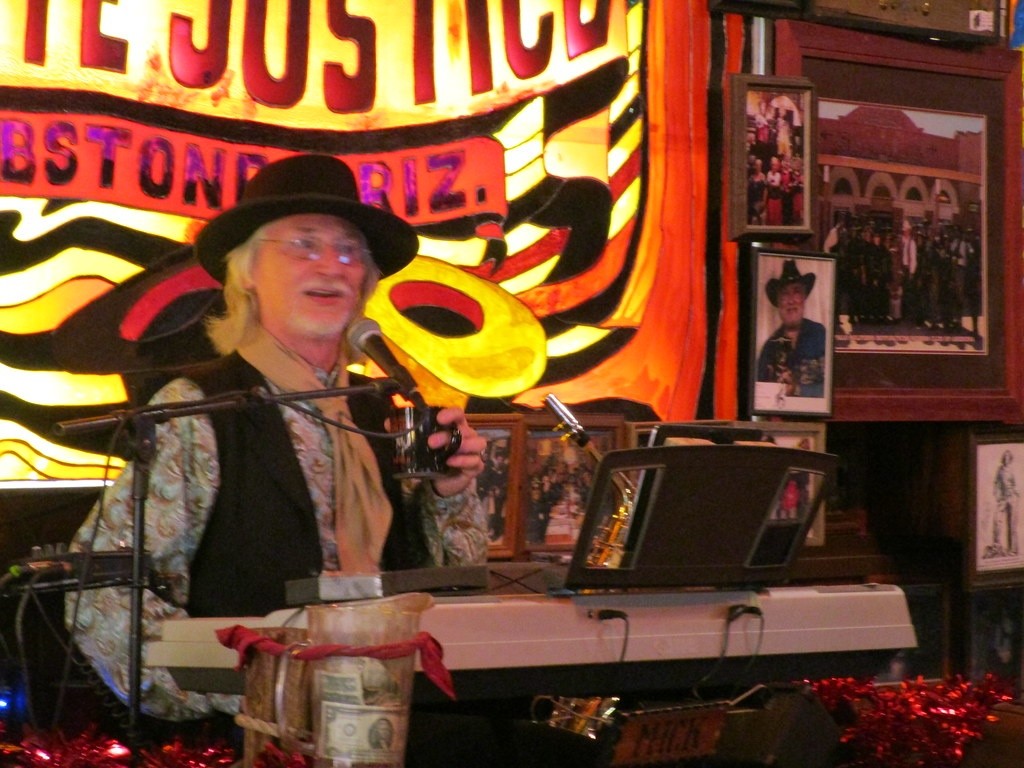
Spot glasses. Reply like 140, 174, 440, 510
257, 234, 367, 265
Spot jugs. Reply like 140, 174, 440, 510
305, 591, 436, 768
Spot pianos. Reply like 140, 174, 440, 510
141, 581, 920, 705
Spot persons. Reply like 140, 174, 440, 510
758, 259, 825, 399
476, 446, 509, 541
764, 435, 810, 519
66, 154, 487, 722
527, 436, 617, 548
745, 99, 804, 227
823, 206, 982, 338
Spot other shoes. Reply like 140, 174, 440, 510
834, 314, 963, 334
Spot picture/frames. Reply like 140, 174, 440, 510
732, 420, 825, 546
968, 432, 1024, 592
744, 246, 846, 419
519, 412, 627, 559
388, 412, 524, 562
892, 570, 948, 678
773, 20, 1024, 426
726, 75, 816, 244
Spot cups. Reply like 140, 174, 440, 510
405, 406, 461, 478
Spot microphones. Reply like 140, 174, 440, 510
345, 317, 427, 415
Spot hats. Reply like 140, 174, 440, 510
901, 221, 911, 233
193, 154, 419, 284
766, 258, 818, 306
